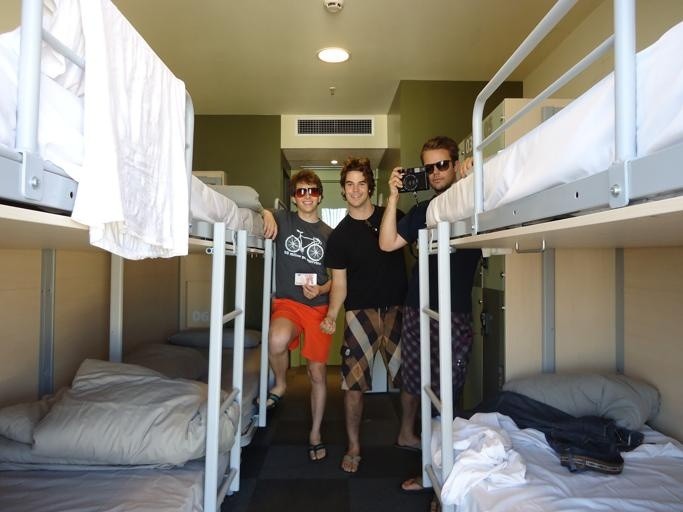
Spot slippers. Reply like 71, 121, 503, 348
339, 448, 362, 476
306, 441, 327, 463
393, 439, 422, 455
399, 475, 424, 494
253, 389, 284, 413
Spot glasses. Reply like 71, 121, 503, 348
424, 159, 452, 173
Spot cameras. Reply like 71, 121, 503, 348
399, 167, 429, 193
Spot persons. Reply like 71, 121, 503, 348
321, 153, 409, 476
250, 166, 336, 463
376, 134, 481, 497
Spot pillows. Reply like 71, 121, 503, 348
500, 370, 660, 434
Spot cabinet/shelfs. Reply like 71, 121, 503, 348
449, 98, 615, 417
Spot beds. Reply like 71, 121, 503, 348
1, 0, 281, 511
412, 0, 682, 512
